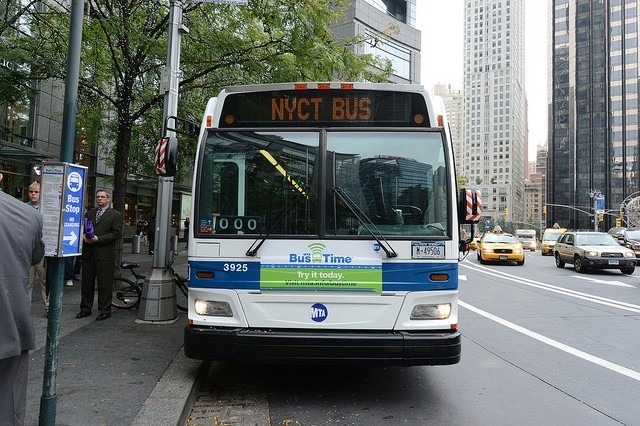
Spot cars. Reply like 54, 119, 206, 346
626, 229, 640, 267
466, 241, 478, 250
609, 227, 625, 244
541, 228, 568, 256
477, 231, 524, 266
554, 231, 638, 275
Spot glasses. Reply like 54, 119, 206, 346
28, 189, 39, 194
97, 195, 109, 198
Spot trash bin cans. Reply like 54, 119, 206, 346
170, 235, 178, 250
132, 235, 140, 254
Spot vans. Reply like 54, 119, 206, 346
515, 229, 536, 252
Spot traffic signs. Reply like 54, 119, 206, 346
596, 196, 605, 214
58, 162, 88, 257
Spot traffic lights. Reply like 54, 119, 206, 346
505, 208, 509, 216
620, 212, 624, 219
616, 218, 621, 226
599, 214, 604, 221
543, 206, 546, 213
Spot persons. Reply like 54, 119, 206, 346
77, 188, 120, 320
183, 217, 189, 249
147, 215, 155, 255
25, 182, 51, 305
589, 72, 612, 94
0, 167, 45, 426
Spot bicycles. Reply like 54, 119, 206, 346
110, 253, 188, 312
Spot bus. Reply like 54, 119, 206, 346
153, 82, 484, 368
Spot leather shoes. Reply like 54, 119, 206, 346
76, 312, 90, 317
96, 313, 111, 320
